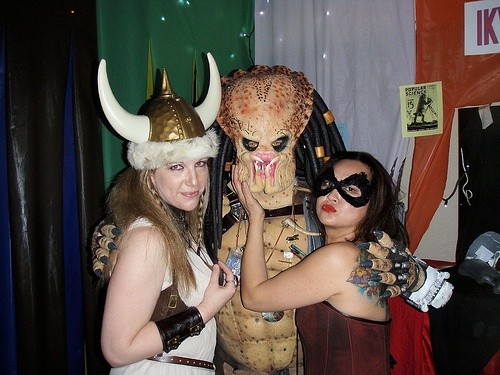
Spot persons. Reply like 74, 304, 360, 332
92, 64, 426, 375
101, 95, 241, 375
229, 151, 410, 375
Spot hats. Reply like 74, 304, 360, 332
98, 51, 222, 170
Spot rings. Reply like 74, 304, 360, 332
224, 275, 238, 287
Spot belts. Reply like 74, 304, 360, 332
146, 356, 214, 370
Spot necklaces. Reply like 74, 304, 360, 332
174, 210, 211, 269
